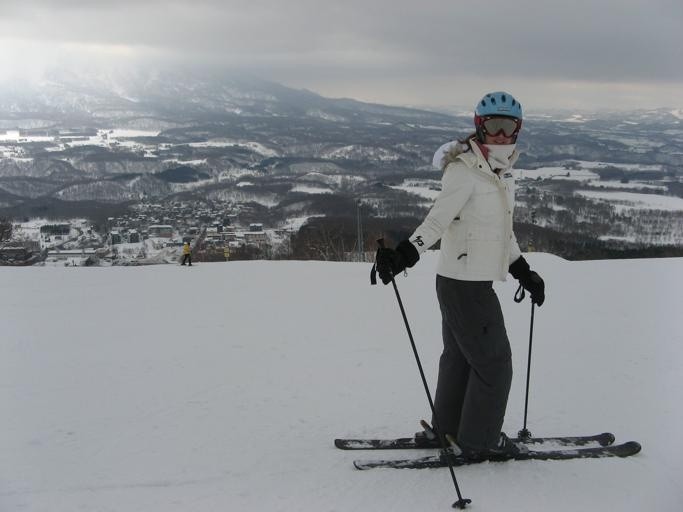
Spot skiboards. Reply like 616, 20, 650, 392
334, 433, 642, 471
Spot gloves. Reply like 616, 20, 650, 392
374, 240, 419, 285
508, 256, 545, 306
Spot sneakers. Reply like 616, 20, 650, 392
457, 432, 521, 458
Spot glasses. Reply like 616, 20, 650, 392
481, 117, 517, 137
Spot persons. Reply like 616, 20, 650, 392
369, 90, 548, 462
180, 242, 194, 267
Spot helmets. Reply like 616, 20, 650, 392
473, 91, 522, 121
